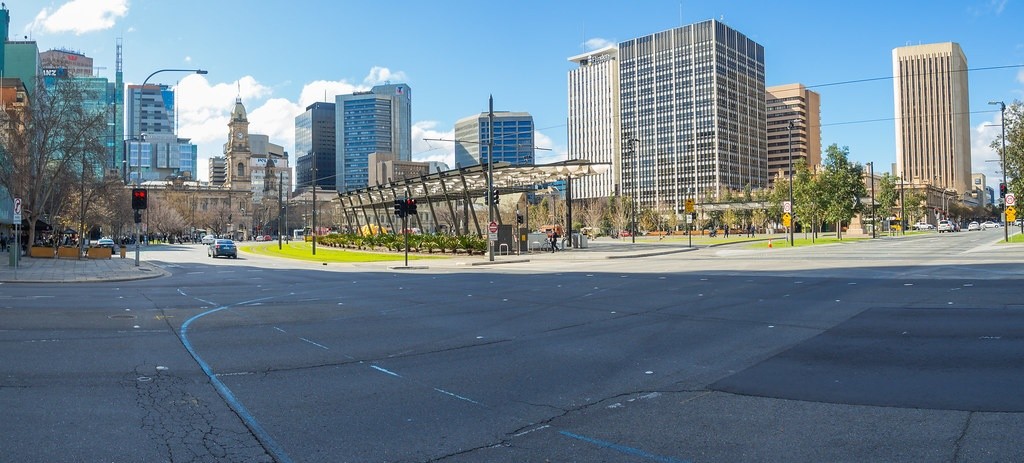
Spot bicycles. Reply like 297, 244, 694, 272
542, 239, 560, 252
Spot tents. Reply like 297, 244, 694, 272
13, 216, 52, 231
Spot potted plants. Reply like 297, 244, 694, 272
119, 244, 127, 258
57, 245, 80, 259
88, 245, 111, 259
30, 243, 55, 258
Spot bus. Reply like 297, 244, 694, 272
294, 226, 328, 242
881, 217, 908, 231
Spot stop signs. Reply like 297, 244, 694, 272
488, 222, 498, 232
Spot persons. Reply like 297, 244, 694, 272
723, 225, 728, 238
0, 232, 15, 252
47, 233, 53, 243
164, 235, 167, 243
120, 240, 127, 258
751, 226, 755, 236
64, 235, 67, 245
177, 236, 188, 244
551, 229, 559, 253
125, 235, 136, 244
148, 235, 154, 244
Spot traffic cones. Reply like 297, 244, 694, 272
767, 239, 772, 248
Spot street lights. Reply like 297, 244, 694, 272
627, 138, 640, 243
988, 100, 1008, 242
135, 69, 207, 265
787, 120, 795, 246
82, 133, 147, 245
866, 162, 905, 238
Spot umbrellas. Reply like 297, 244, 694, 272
64, 228, 77, 239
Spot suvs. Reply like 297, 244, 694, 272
938, 220, 954, 232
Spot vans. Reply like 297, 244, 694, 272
539, 224, 562, 236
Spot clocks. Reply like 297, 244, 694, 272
236, 132, 244, 139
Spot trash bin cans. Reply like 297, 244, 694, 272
169, 238, 174, 244
580, 233, 588, 248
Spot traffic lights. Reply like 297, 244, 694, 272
1000, 183, 1007, 198
394, 198, 417, 217
483, 190, 488, 204
132, 188, 147, 223
493, 189, 499, 204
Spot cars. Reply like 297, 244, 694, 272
256, 235, 293, 242
954, 219, 1024, 231
202, 235, 237, 258
912, 222, 933, 229
89, 239, 115, 255
